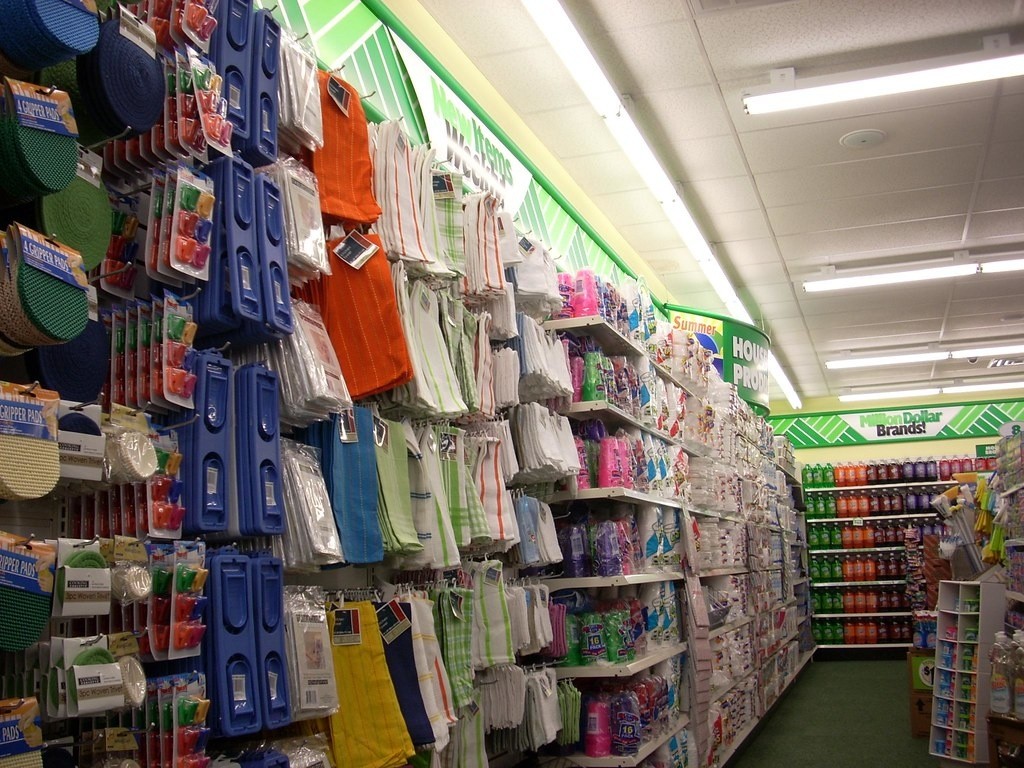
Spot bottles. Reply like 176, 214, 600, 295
802, 453, 997, 644
989, 630, 1012, 713
1012, 630, 1024, 721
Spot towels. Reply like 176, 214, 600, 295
314, 53, 581, 767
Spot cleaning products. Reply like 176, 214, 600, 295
990, 629, 1024, 722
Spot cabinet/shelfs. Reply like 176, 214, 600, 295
998, 482, 1024, 603
542, 314, 1004, 768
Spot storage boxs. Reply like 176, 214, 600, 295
996, 431, 1024, 629
907, 651, 935, 740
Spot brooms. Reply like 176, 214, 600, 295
934, 546, 978, 614
